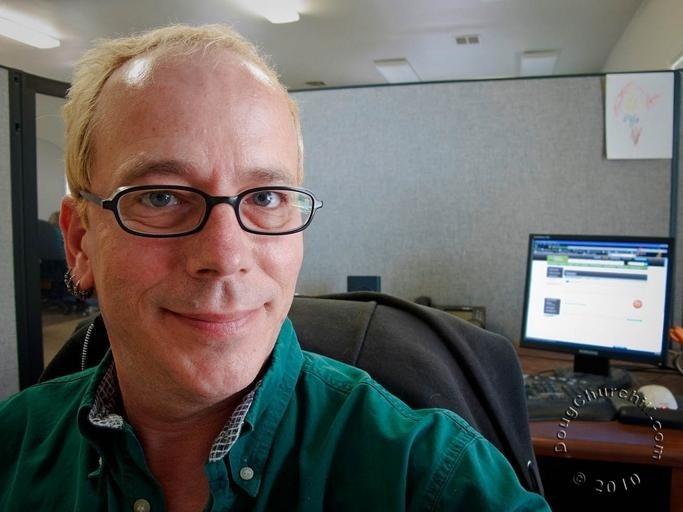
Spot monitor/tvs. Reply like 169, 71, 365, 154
521, 233, 675, 389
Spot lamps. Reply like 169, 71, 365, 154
249, 1, 300, 25
520, 50, 557, 76
0, 18, 60, 49
373, 59, 422, 84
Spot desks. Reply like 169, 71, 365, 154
510, 345, 682, 512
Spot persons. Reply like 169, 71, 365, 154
0, 21, 553, 512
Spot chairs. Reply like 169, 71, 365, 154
34, 290, 542, 497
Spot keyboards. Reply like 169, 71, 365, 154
524, 372, 616, 420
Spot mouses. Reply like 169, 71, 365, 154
637, 384, 678, 413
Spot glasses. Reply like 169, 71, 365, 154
79, 184, 324, 236
